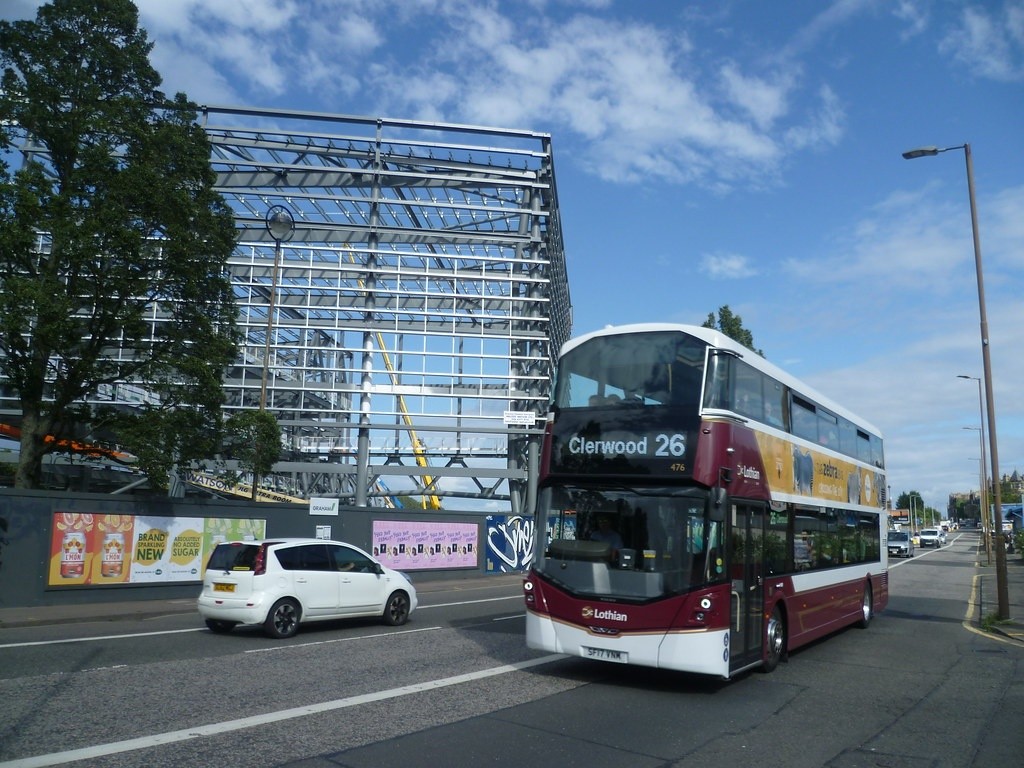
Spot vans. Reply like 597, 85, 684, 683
888, 530, 915, 558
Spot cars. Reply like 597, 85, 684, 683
196, 538, 419, 638
935, 520, 967, 545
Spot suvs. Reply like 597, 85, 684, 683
919, 529, 942, 547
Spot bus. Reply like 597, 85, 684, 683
520, 322, 889, 684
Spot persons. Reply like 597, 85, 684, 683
591, 514, 623, 560
588, 394, 620, 409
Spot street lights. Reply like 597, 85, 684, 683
901, 143, 1011, 621
251, 205, 298, 501
909, 494, 915, 535
962, 426, 988, 554
956, 374, 993, 565
914, 496, 921, 534
